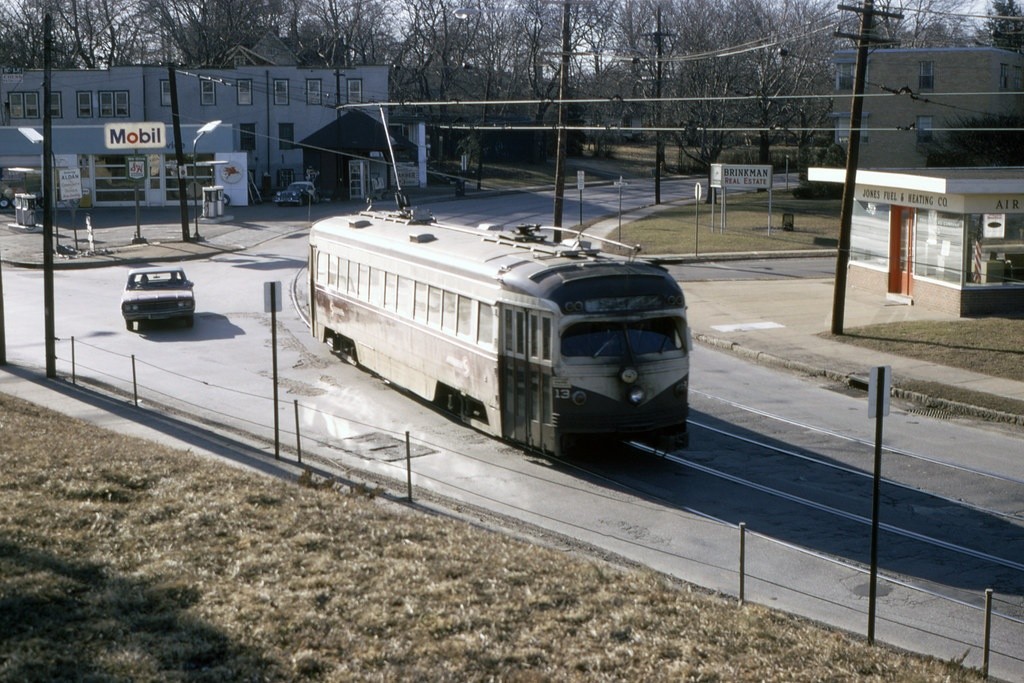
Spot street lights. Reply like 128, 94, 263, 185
457, 7, 571, 244
464, 65, 491, 192
18, 127, 63, 254
194, 119, 224, 240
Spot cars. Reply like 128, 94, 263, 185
274, 182, 319, 206
121, 266, 196, 329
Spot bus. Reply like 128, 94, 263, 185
312, 208, 692, 458
